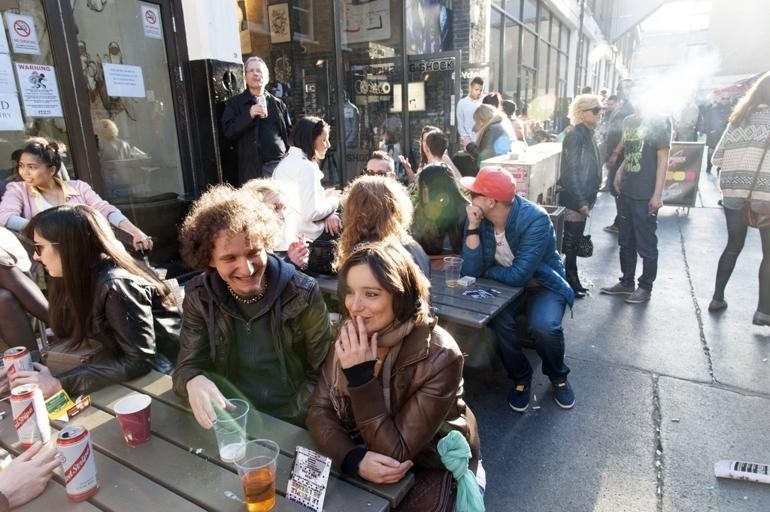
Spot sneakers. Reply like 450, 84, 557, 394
599, 184, 611, 192
553, 379, 575, 409
604, 224, 619, 233
601, 281, 634, 295
624, 286, 651, 304
508, 382, 531, 412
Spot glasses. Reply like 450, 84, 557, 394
366, 170, 387, 177
33, 243, 60, 256
582, 107, 601, 115
470, 191, 481, 199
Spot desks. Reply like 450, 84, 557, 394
1, 332, 414, 510
479, 142, 563, 205
306, 263, 526, 392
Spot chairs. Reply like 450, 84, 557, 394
1, 226, 158, 356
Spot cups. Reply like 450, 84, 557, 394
212, 398, 250, 464
233, 437, 279, 512
442, 255, 464, 288
112, 392, 155, 449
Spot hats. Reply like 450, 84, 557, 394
459, 167, 516, 202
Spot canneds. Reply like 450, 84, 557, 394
256, 95, 268, 119
3, 346, 34, 391
56, 425, 99, 503
9, 384, 52, 451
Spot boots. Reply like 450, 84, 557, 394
567, 266, 589, 291
567, 276, 585, 298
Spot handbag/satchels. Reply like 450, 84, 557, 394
0, 225, 32, 274
562, 235, 593, 257
741, 200, 770, 229
391, 468, 456, 512
309, 239, 338, 275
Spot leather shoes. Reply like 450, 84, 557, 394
753, 311, 770, 325
709, 299, 728, 311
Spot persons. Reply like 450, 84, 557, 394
708, 73, 770, 326
0, 56, 575, 511
674, 90, 744, 177
559, 74, 673, 303
